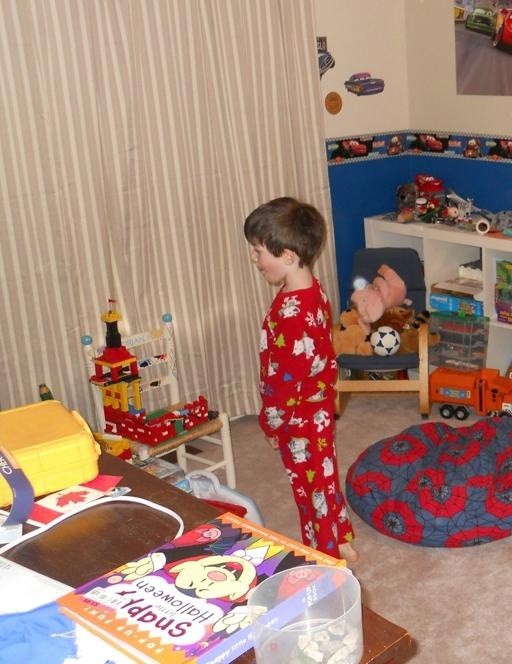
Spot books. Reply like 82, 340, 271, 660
59, 511, 350, 664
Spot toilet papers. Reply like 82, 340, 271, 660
471, 213, 490, 235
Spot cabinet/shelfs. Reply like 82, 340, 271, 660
363, 217, 512, 331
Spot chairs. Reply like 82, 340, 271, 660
331, 248, 430, 419
82, 312, 236, 490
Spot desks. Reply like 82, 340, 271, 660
0, 452, 414, 663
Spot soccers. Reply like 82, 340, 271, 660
371, 325, 400, 358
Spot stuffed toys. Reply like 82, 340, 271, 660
331, 260, 441, 360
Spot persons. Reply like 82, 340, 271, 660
243, 196, 359, 569
